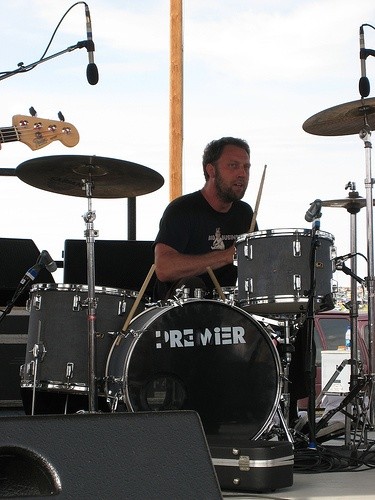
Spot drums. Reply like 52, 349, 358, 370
102, 299, 282, 441
234, 227, 337, 316
177, 285, 299, 344
17, 283, 151, 398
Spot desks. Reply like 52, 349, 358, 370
321, 350, 351, 393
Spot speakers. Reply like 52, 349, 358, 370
63, 239, 156, 298
0, 238, 55, 306
0, 410, 223, 500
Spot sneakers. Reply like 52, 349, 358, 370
295, 415, 345, 440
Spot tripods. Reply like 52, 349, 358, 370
286, 96, 375, 465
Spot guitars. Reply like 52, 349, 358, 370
0, 107, 80, 151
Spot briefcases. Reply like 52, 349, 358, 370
207, 438, 295, 491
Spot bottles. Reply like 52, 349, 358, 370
345, 326, 351, 351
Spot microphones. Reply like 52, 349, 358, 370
41, 250, 57, 272
359, 24, 370, 97
84, 4, 99, 85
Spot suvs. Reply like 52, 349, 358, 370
298, 310, 369, 412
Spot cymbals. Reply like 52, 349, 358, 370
310, 191, 375, 209
15, 154, 164, 199
302, 95, 375, 137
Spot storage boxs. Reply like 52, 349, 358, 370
208, 439, 294, 494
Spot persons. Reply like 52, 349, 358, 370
152, 137, 346, 443
